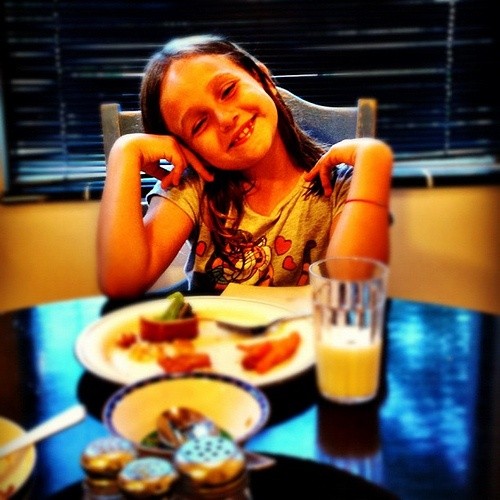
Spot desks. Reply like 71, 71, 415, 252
0, 294, 500, 500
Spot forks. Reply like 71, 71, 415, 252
216, 310, 312, 339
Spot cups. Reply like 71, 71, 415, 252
306, 256, 389, 405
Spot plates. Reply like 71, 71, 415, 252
74, 295, 316, 389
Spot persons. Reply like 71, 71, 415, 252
95, 34, 393, 301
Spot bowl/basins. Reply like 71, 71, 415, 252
0, 415, 38, 500
102, 371, 271, 457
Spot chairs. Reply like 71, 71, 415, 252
100, 85, 378, 168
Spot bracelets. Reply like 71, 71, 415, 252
344, 199, 388, 211
